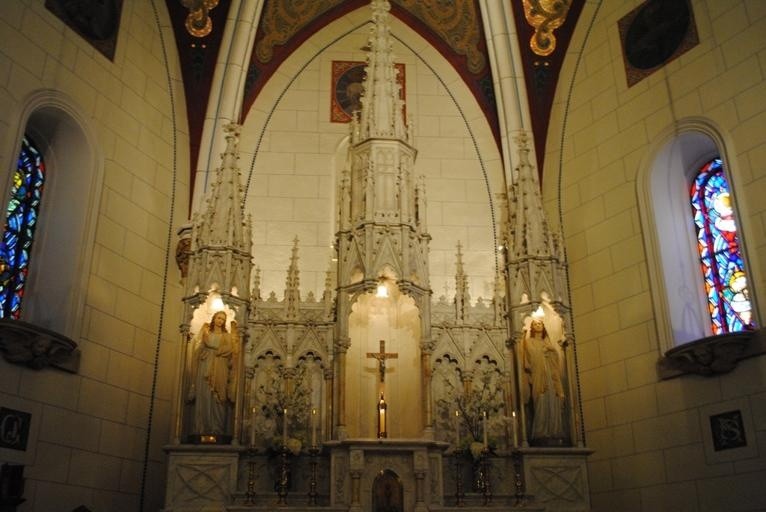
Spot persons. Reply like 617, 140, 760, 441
523, 319, 568, 440
188, 312, 234, 434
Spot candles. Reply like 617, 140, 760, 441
483, 411, 488, 449
283, 408, 287, 447
512, 412, 517, 448
311, 408, 315, 447
379, 392, 386, 434
455, 410, 460, 449
250, 407, 255, 446
